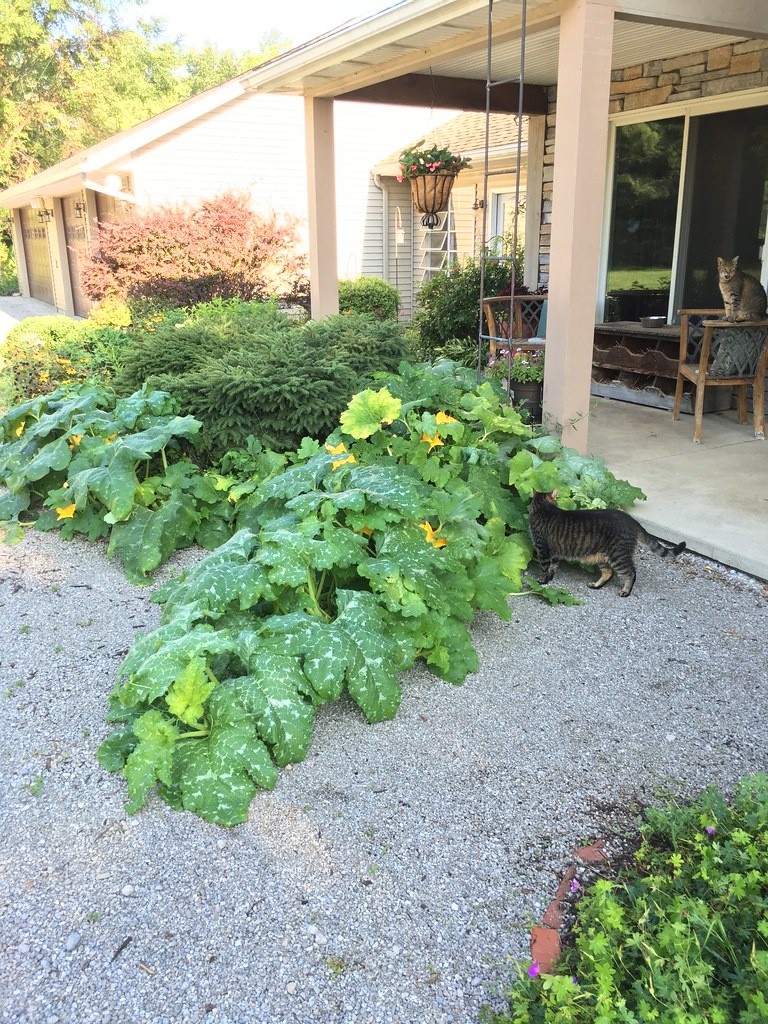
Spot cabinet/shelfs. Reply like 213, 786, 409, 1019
589, 321, 735, 414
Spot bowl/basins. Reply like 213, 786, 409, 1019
638, 316, 667, 327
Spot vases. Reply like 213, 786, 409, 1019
509, 381, 543, 424
407, 169, 456, 229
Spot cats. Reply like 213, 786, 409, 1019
716, 255, 768, 323
527, 487, 688, 599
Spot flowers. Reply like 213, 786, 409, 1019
482, 346, 544, 385
396, 139, 473, 184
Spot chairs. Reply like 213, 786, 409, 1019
477, 294, 548, 364
673, 309, 768, 444
